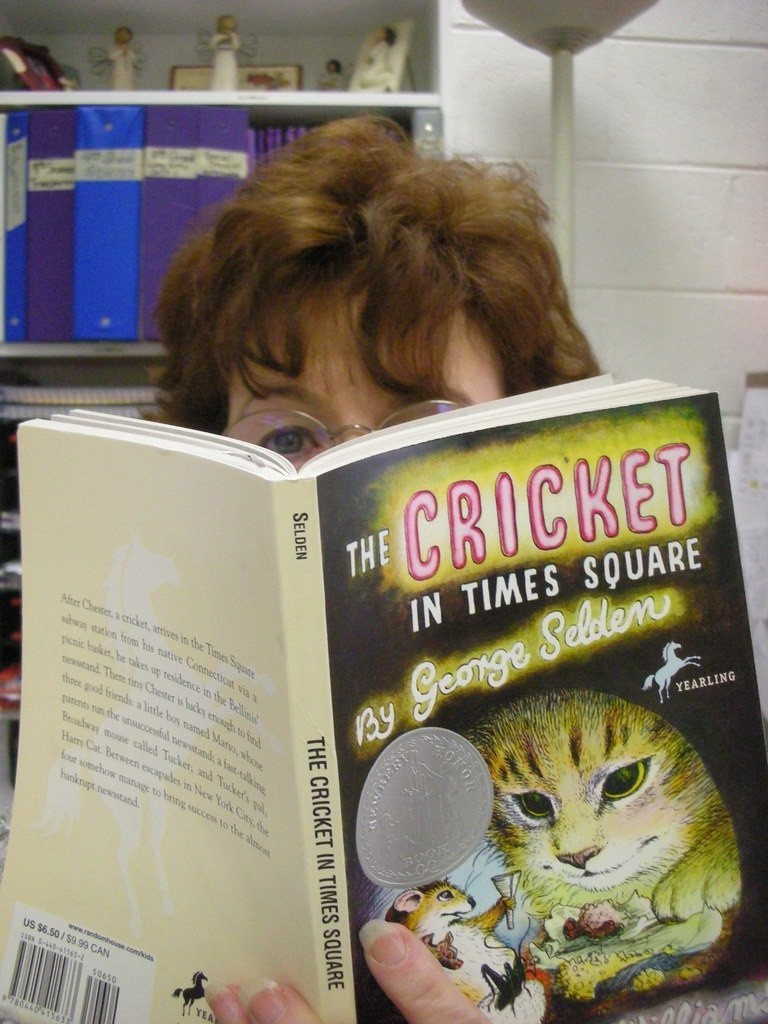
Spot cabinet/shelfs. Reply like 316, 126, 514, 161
0, 0, 453, 858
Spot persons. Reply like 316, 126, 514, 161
143, 118, 601, 1024
359, 25, 395, 90
208, 15, 241, 90
109, 28, 144, 88
318, 60, 345, 89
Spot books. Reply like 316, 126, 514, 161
0, 373, 768, 1024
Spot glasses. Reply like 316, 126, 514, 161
221, 398, 464, 474
